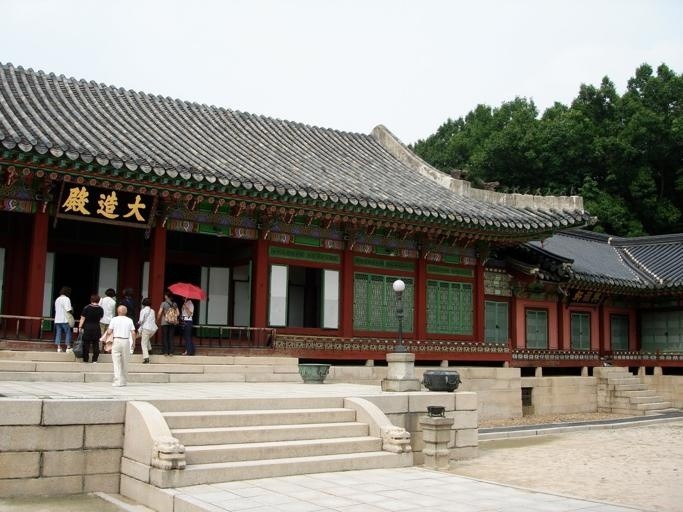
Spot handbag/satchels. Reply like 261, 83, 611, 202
65, 312, 75, 327
71, 339, 84, 357
163, 299, 179, 324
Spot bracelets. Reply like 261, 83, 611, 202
78, 327, 82, 329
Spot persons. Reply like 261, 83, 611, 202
119, 287, 137, 352
136, 297, 157, 362
104, 304, 136, 386
77, 293, 103, 362
154, 291, 179, 355
179, 299, 195, 355
98, 287, 116, 354
53, 286, 75, 354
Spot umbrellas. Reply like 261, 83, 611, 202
167, 281, 207, 302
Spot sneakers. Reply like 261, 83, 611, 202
142, 349, 195, 364
111, 380, 126, 386
56, 347, 75, 354
